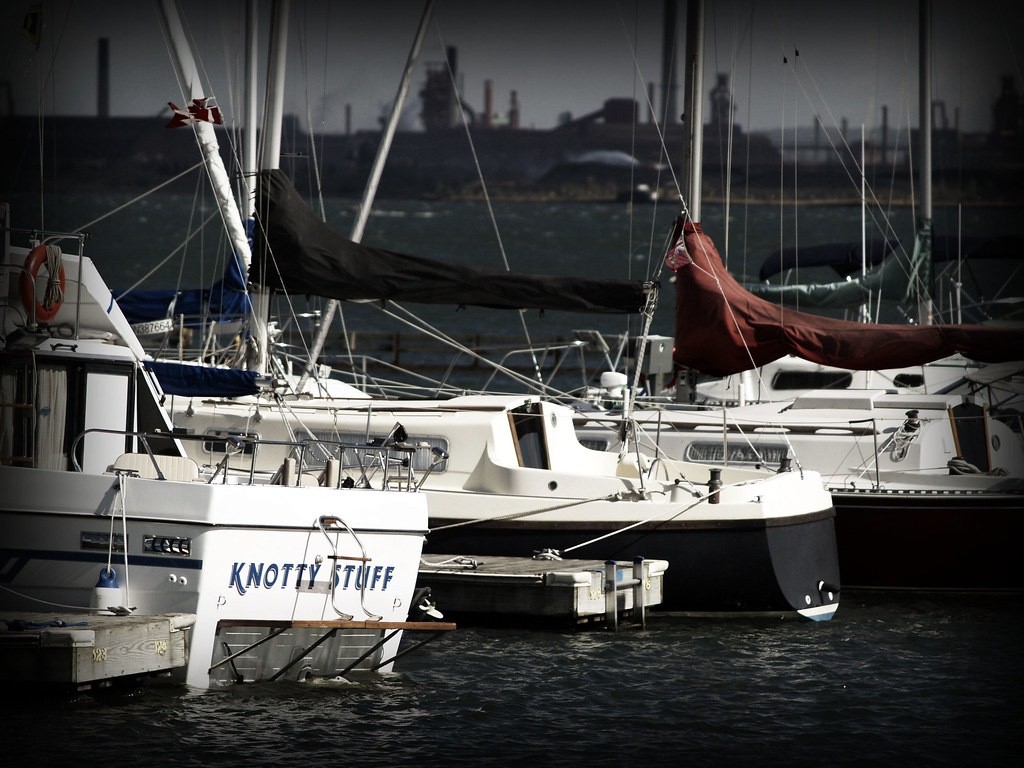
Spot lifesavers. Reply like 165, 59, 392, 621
20, 245, 66, 321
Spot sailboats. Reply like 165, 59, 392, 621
0, 0, 1024, 702
